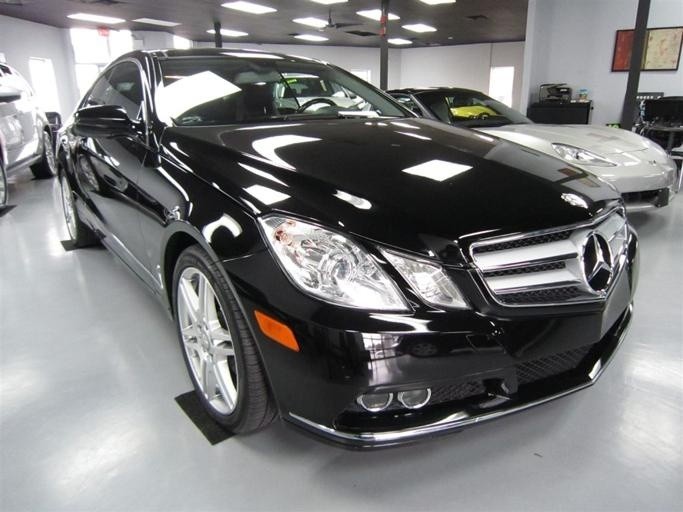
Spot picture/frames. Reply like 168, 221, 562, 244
611, 26, 683, 72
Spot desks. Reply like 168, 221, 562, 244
527, 100, 592, 124
633, 96, 683, 170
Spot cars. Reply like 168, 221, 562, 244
50, 46, 643, 455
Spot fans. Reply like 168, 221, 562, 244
318, 10, 365, 31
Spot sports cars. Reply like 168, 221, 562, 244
383, 82, 680, 217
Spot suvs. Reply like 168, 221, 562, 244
0, 58, 58, 212
